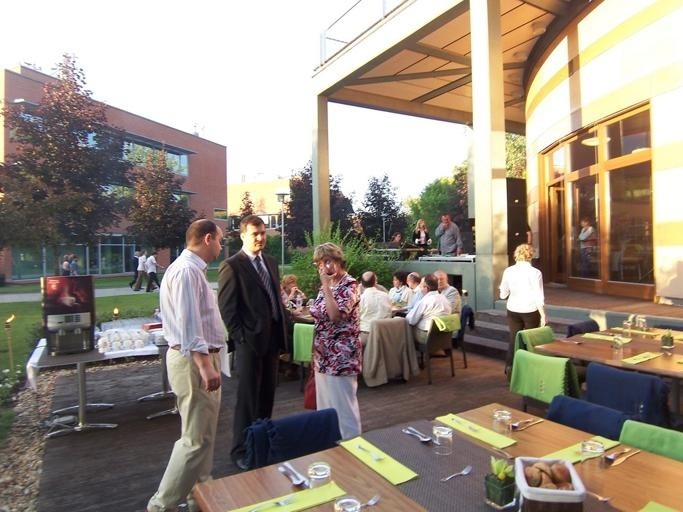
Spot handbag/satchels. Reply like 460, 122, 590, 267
304, 369, 315, 410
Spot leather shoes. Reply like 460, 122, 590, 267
173, 503, 189, 512
235, 458, 248, 470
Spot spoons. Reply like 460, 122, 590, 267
401, 429, 432, 443
277, 466, 304, 487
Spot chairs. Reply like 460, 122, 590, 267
617, 243, 643, 281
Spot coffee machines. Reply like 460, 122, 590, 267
39, 275, 94, 356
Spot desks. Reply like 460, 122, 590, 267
98, 318, 181, 421
27, 334, 162, 437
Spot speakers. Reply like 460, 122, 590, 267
506, 178, 529, 233
508, 233, 528, 265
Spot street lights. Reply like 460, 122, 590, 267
381, 214, 388, 244
276, 192, 290, 272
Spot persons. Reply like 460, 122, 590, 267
146, 219, 232, 512
218, 214, 288, 470
498, 242, 546, 383
131, 249, 148, 291
385, 231, 408, 260
412, 218, 432, 259
128, 251, 143, 289
577, 216, 596, 277
308, 242, 362, 441
280, 274, 308, 312
140, 250, 166, 293
434, 214, 463, 256
358, 270, 462, 345
62, 254, 79, 276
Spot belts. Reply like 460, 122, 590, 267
171, 344, 220, 353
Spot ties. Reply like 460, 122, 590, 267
254, 255, 279, 321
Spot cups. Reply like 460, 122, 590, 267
492, 409, 512, 457
333, 495, 361, 512
431, 423, 453, 456
324, 262, 335, 275
95, 328, 166, 353
608, 315, 646, 363
578, 438, 606, 501
307, 461, 332, 501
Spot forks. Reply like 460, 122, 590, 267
438, 466, 472, 483
359, 495, 380, 509
246, 493, 294, 512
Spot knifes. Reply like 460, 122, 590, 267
283, 462, 311, 490
513, 419, 544, 432
407, 426, 440, 446
608, 449, 640, 467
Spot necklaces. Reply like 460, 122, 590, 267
329, 272, 348, 293
